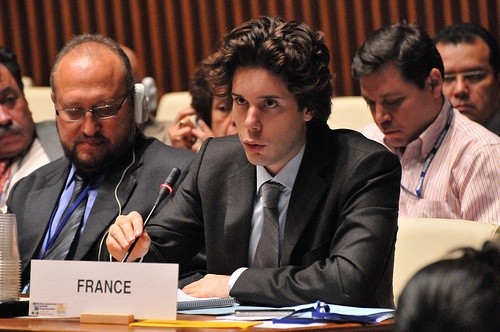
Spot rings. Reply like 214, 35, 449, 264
179, 120, 184, 126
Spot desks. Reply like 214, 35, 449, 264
0, 295, 395, 332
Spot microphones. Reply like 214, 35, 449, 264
121, 167, 181, 262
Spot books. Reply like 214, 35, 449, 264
176, 288, 234, 308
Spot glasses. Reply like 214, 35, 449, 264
443, 69, 496, 87
55, 92, 130, 122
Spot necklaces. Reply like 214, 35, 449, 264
27, 166, 110, 293
399, 104, 454, 199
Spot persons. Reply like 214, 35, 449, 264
0, 45, 64, 214
168, 52, 239, 154
6, 35, 197, 295
432, 21, 500, 136
106, 15, 402, 309
352, 21, 500, 224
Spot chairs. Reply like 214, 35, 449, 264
155, 91, 191, 121
327, 96, 373, 130
393, 219, 497, 311
23, 86, 57, 123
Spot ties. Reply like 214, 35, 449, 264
251, 181, 286, 269
0, 158, 12, 196
25, 170, 99, 295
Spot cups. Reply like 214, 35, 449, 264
0, 213, 23, 303
